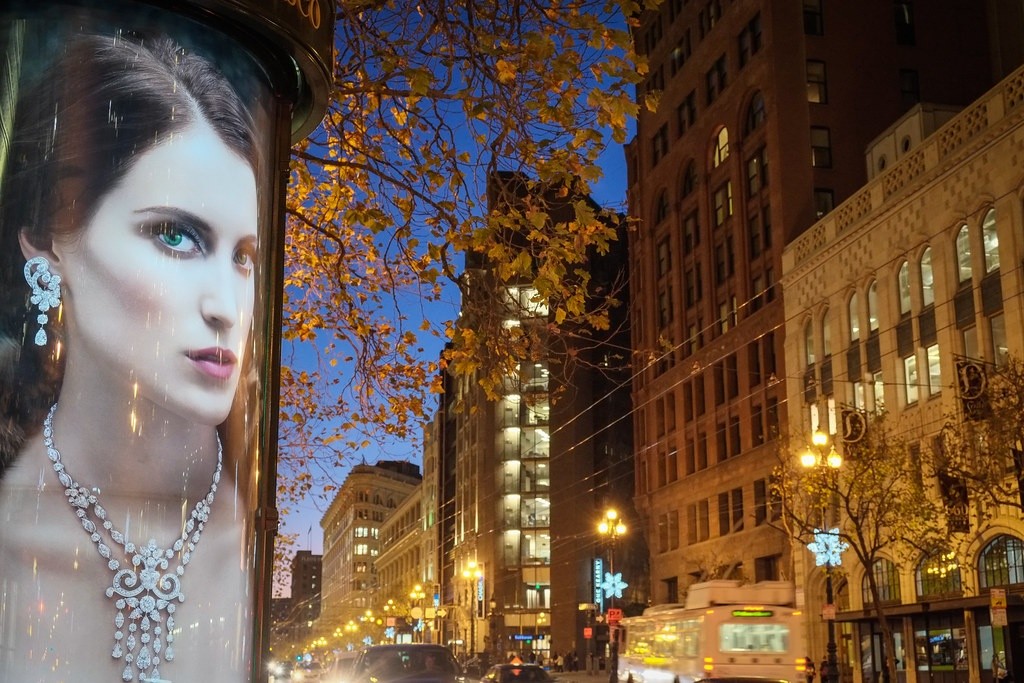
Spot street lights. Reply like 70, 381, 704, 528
463, 559, 483, 659
410, 585, 425, 642
598, 509, 627, 683
800, 426, 841, 682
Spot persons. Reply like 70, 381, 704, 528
508, 650, 579, 673
0, 33, 263, 683
424, 655, 439, 672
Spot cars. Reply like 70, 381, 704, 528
480, 663, 552, 683
292, 660, 326, 682
274, 660, 293, 679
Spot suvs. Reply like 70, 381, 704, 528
319, 654, 358, 682
346, 643, 467, 683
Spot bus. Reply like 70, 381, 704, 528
617, 577, 808, 683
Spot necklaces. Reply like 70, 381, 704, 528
43, 399, 224, 683
